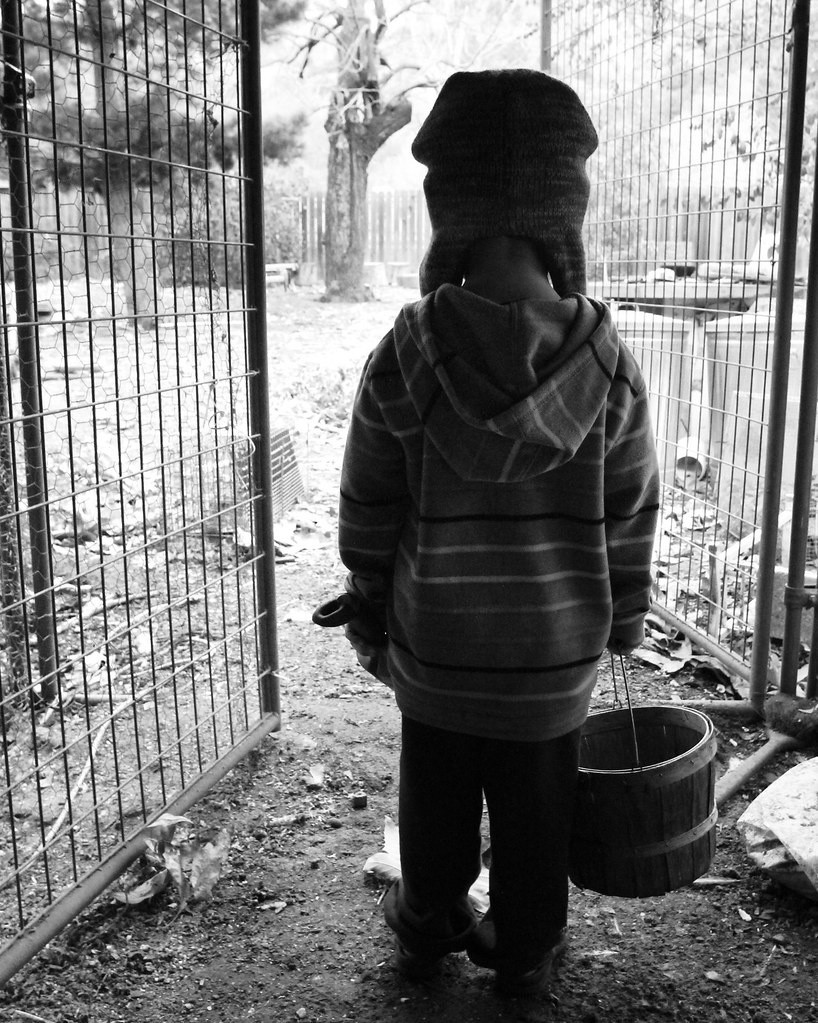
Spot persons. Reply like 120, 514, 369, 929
313, 69, 660, 977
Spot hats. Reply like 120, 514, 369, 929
413, 69, 600, 300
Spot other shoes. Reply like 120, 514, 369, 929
494, 935, 564, 992
397, 943, 447, 980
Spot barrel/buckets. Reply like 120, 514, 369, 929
567, 653, 718, 897
